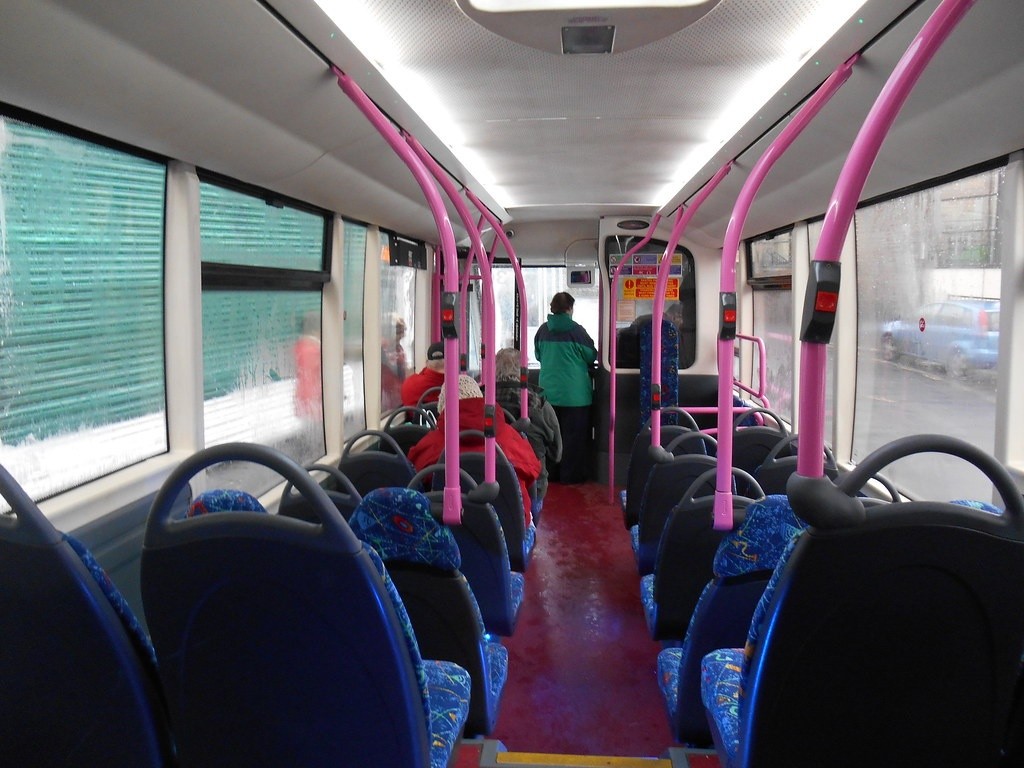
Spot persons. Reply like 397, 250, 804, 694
379, 313, 407, 410
629, 304, 684, 329
401, 342, 445, 417
535, 292, 598, 485
407, 375, 541, 530
479, 348, 563, 499
294, 311, 322, 420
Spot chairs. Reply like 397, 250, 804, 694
732, 409, 792, 495
0, 463, 177, 768
631, 432, 737, 575
701, 433, 1024, 768
413, 386, 442, 431
278, 465, 360, 526
621, 407, 707, 530
337, 429, 422, 499
502, 408, 542, 525
658, 494, 808, 748
833, 473, 900, 508
141, 442, 472, 768
183, 488, 267, 516
378, 407, 437, 458
744, 436, 840, 496
431, 431, 535, 573
640, 466, 767, 641
405, 466, 526, 636
346, 487, 509, 740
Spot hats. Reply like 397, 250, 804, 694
427, 342, 444, 361
438, 374, 483, 414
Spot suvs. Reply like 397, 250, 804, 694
881, 296, 1000, 378
